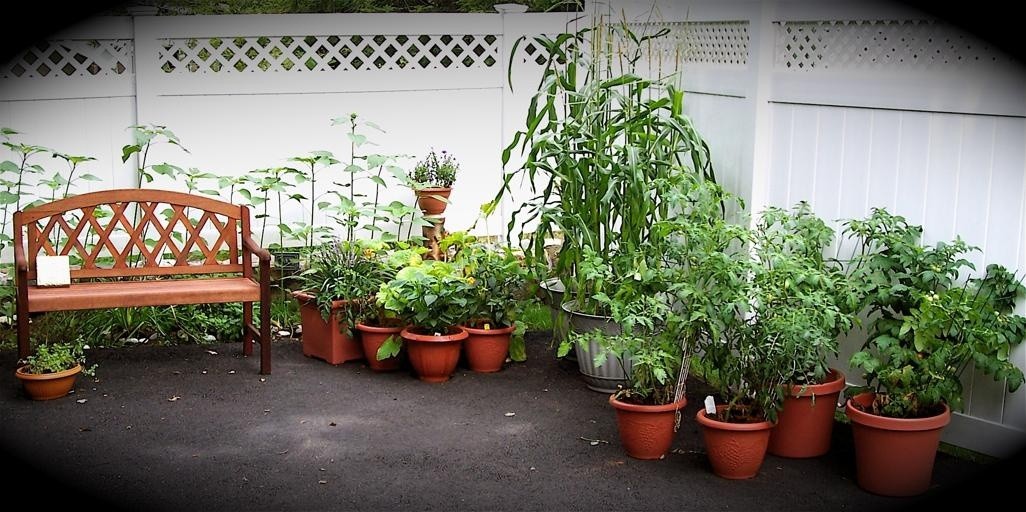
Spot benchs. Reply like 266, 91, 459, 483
12, 188, 271, 376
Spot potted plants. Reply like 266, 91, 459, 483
277, 114, 537, 379
14, 342, 82, 402
536, 2, 1026, 497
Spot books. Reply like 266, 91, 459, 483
34, 254, 71, 288
